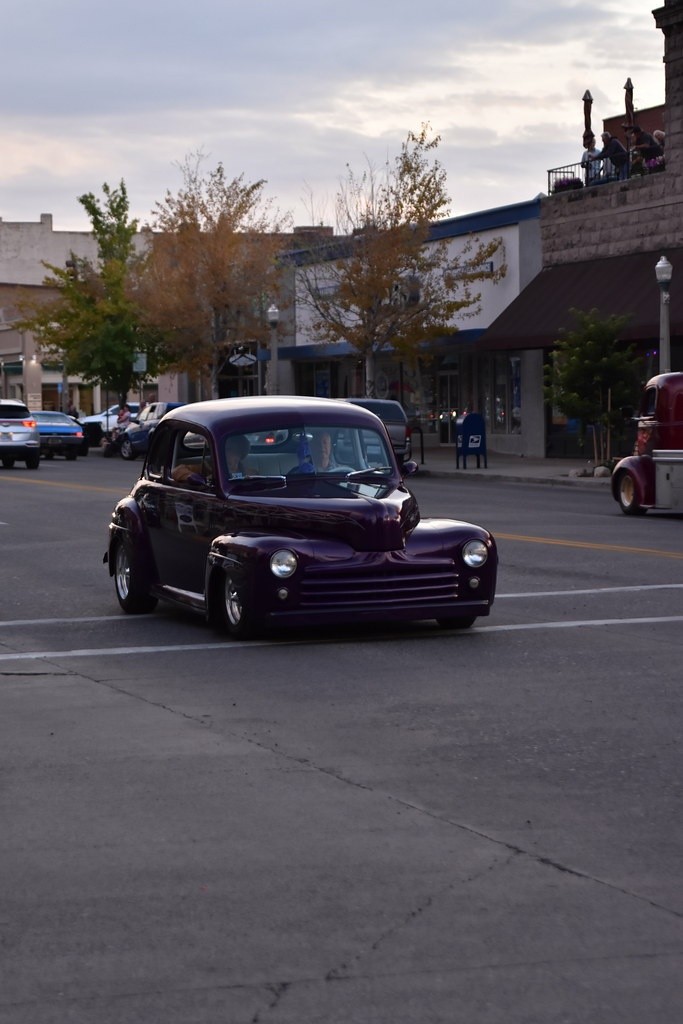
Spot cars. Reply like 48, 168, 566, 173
610, 370, 683, 517
102, 396, 500, 643
325, 396, 419, 483
117, 402, 191, 461
29, 410, 85, 461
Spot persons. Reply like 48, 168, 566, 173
67, 406, 79, 419
117, 405, 130, 436
581, 126, 666, 185
172, 434, 259, 484
137, 392, 155, 417
288, 430, 346, 473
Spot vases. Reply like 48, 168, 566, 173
552, 182, 583, 193
648, 164, 665, 174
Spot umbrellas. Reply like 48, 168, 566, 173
582, 90, 595, 187
622, 78, 634, 179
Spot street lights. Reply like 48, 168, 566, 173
267, 303, 280, 395
656, 255, 674, 375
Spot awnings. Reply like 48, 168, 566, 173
479, 245, 683, 352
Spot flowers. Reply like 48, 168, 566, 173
554, 176, 580, 188
646, 154, 664, 168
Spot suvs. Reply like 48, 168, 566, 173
74, 402, 141, 448
0, 397, 42, 471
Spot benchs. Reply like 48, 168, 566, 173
173, 453, 301, 477
